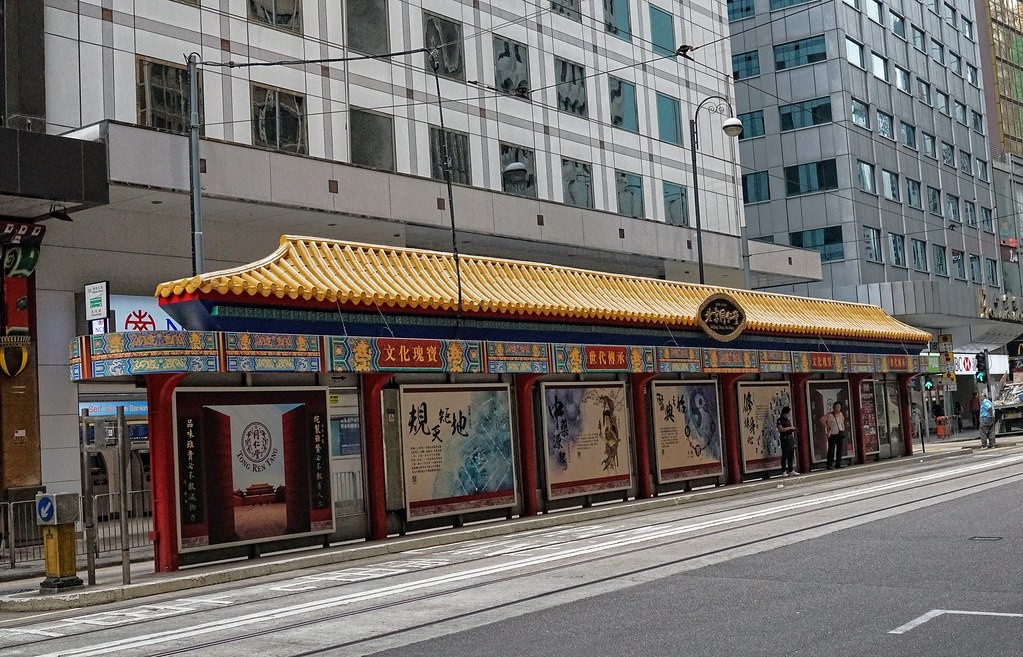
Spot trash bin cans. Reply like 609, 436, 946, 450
936, 416, 949, 439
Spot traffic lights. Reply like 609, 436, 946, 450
925, 375, 933, 390
976, 352, 988, 383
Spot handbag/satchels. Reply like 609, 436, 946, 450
838, 431, 846, 437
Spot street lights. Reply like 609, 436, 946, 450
689, 96, 743, 285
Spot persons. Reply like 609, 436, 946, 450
934, 405, 943, 425
955, 402, 964, 433
777, 406, 800, 477
911, 403, 922, 437
978, 393, 996, 449
970, 393, 980, 429
821, 401, 845, 469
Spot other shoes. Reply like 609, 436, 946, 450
979, 446, 996, 449
827, 465, 844, 469
783, 470, 800, 476
